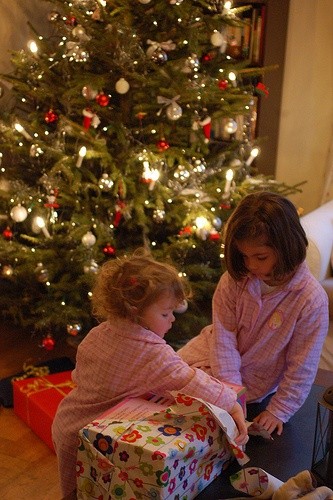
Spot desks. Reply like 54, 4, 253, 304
193, 382, 328, 500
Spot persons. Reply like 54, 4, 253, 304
51, 247, 250, 500
175, 192, 329, 436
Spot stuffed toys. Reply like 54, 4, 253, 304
272, 469, 333, 500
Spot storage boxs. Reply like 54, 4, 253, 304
75, 383, 246, 500
13, 370, 75, 454
